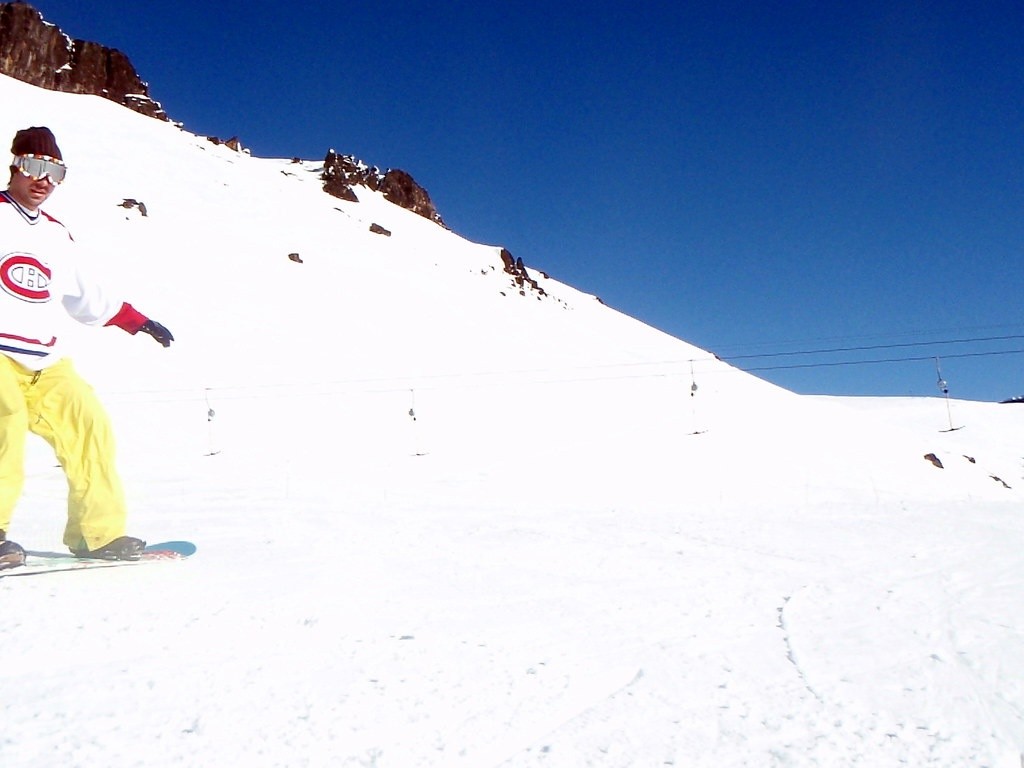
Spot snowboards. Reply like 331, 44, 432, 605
0, 540, 196, 577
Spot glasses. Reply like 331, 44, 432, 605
10, 155, 67, 186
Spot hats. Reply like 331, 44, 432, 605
10, 127, 62, 161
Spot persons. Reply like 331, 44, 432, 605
0, 126, 175, 568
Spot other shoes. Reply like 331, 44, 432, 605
68, 536, 146, 562
0, 529, 28, 573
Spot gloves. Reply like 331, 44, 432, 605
138, 320, 175, 348
